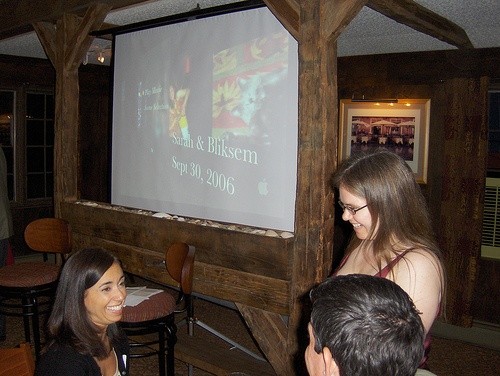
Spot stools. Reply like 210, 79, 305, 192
117, 241, 196, 376
0, 216, 75, 356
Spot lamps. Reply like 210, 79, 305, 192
95, 49, 105, 64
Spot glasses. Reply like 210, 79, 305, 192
338, 200, 374, 215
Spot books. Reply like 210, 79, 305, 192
121, 286, 164, 307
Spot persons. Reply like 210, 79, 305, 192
35, 247, 130, 376
0, 146, 14, 269
305, 274, 438, 376
350, 119, 417, 136
167, 83, 191, 141
331, 147, 447, 373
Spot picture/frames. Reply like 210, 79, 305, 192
339, 99, 432, 185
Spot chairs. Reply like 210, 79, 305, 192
0, 339, 36, 376
